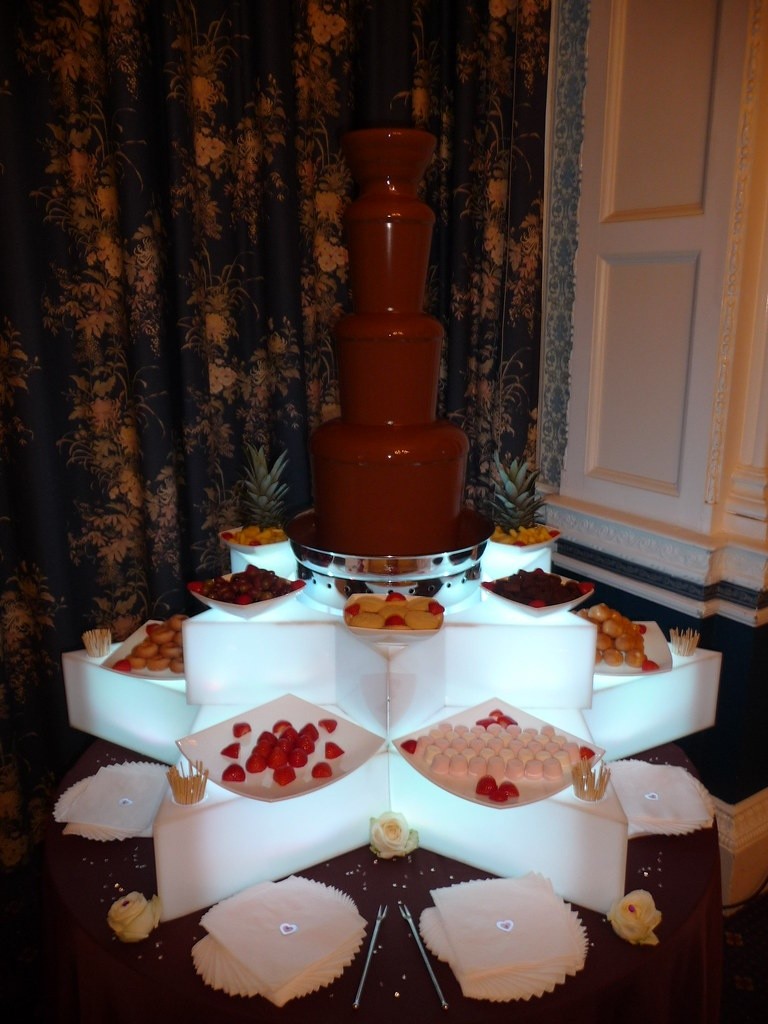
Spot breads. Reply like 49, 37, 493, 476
491, 569, 580, 605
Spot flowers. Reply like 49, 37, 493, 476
607, 889, 662, 945
368, 810, 419, 858
106, 891, 162, 942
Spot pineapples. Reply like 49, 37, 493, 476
350, 594, 438, 630
228, 527, 287, 547
490, 526, 551, 546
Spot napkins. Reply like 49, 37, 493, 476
52, 762, 169, 843
418, 870, 589, 1004
603, 760, 717, 838
191, 874, 368, 1007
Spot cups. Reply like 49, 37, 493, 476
669, 625, 700, 657
165, 765, 208, 804
83, 631, 112, 657
572, 759, 610, 804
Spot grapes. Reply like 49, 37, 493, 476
201, 570, 291, 606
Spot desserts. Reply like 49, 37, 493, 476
417, 722, 581, 782
126, 614, 190, 671
575, 602, 648, 668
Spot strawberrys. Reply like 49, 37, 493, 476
534, 568, 545, 573
112, 660, 132, 671
476, 709, 519, 729
529, 599, 545, 607
146, 623, 157, 634
549, 529, 560, 537
221, 532, 233, 540
639, 624, 647, 634
429, 601, 445, 615
401, 740, 417, 754
579, 746, 595, 759
513, 542, 525, 546
475, 775, 519, 803
580, 582, 593, 594
249, 541, 260, 546
385, 592, 406, 601
220, 719, 346, 785
641, 661, 660, 670
482, 582, 495, 591
344, 604, 360, 616
187, 581, 203, 592
291, 580, 306, 591
385, 615, 404, 625
246, 565, 258, 572
235, 594, 251, 605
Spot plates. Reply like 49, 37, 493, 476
342, 593, 445, 641
175, 693, 386, 804
594, 620, 673, 676
189, 571, 307, 620
219, 525, 288, 555
488, 524, 562, 553
479, 571, 594, 616
391, 697, 607, 810
99, 619, 185, 681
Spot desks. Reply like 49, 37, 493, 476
45, 738, 724, 1024
